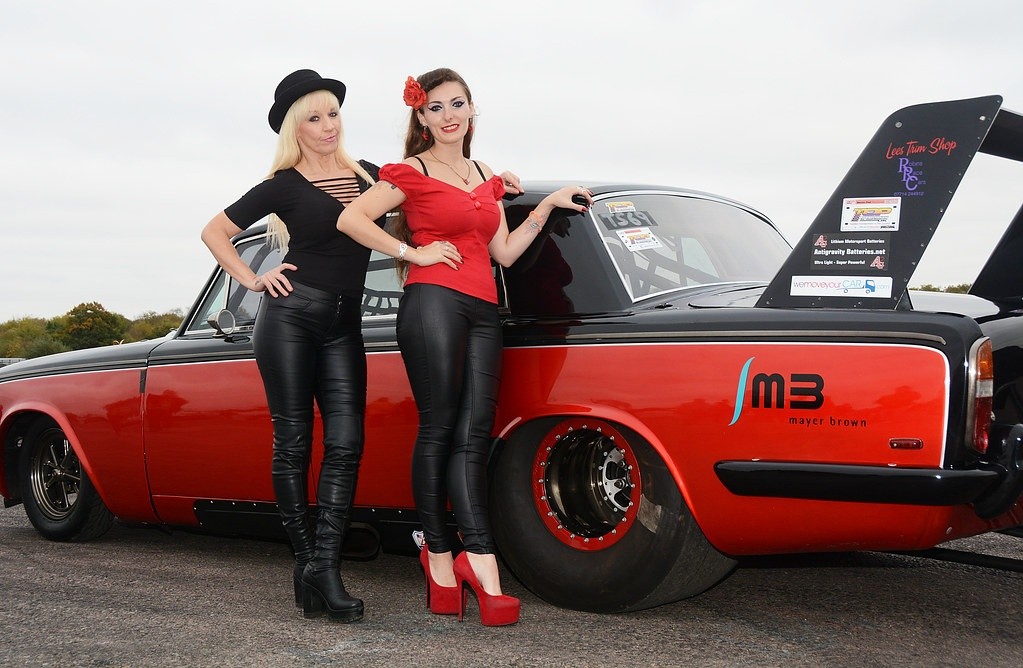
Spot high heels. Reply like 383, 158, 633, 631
419, 544, 466, 614
453, 550, 520, 626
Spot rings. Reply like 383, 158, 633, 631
439, 241, 447, 244
576, 184, 586, 194
446, 246, 449, 252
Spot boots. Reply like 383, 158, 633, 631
279, 512, 350, 607
301, 508, 364, 624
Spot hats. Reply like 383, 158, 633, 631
268, 69, 346, 134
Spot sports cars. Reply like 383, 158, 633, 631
0, 96, 1022, 615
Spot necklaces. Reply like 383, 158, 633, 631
429, 149, 470, 185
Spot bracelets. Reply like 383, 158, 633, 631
529, 211, 542, 233
397, 241, 407, 261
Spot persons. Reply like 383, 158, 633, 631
201, 69, 525, 624
337, 68, 594, 627
